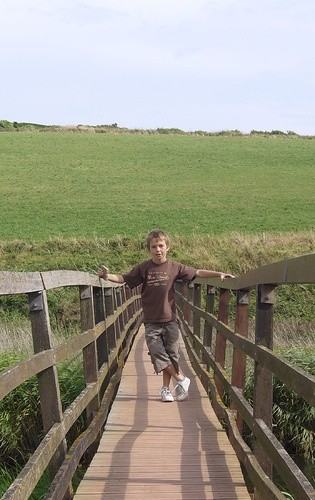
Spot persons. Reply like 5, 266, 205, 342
98, 230, 236, 402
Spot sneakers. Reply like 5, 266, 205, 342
176, 376, 191, 401
161, 386, 174, 402
